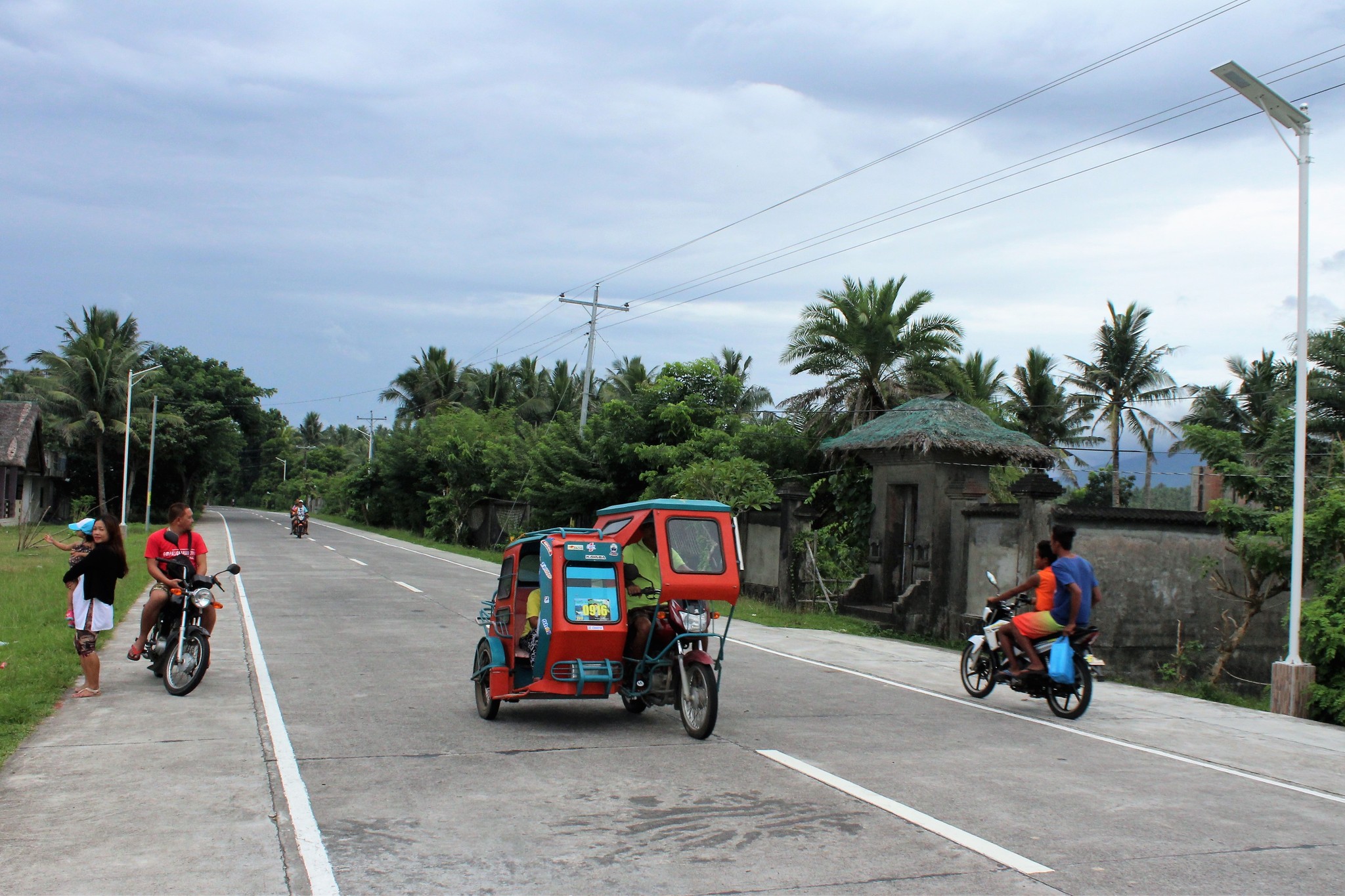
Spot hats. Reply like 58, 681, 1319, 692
299, 500, 303, 503
68, 518, 95, 536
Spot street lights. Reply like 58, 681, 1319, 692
1209, 57, 1321, 718
119, 364, 164, 539
275, 457, 287, 497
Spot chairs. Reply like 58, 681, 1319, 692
515, 587, 535, 659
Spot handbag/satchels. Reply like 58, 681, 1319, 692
1049, 636, 1074, 684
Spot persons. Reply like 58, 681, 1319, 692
63, 514, 129, 698
986, 540, 1058, 677
518, 588, 540, 671
128, 503, 222, 669
622, 511, 694, 692
44, 518, 95, 628
290, 499, 310, 535
1010, 524, 1102, 674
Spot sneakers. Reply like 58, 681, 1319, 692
65, 610, 74, 621
68, 621, 74, 629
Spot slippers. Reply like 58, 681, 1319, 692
996, 670, 1020, 678
1020, 668, 1046, 675
128, 645, 144, 661
197, 655, 209, 669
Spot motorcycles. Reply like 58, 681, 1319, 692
292, 513, 309, 539
135, 531, 242, 697
961, 570, 1106, 721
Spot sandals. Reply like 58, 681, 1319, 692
74, 688, 100, 698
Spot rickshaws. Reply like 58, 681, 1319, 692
468, 497, 746, 743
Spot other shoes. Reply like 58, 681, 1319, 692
305, 532, 308, 535
